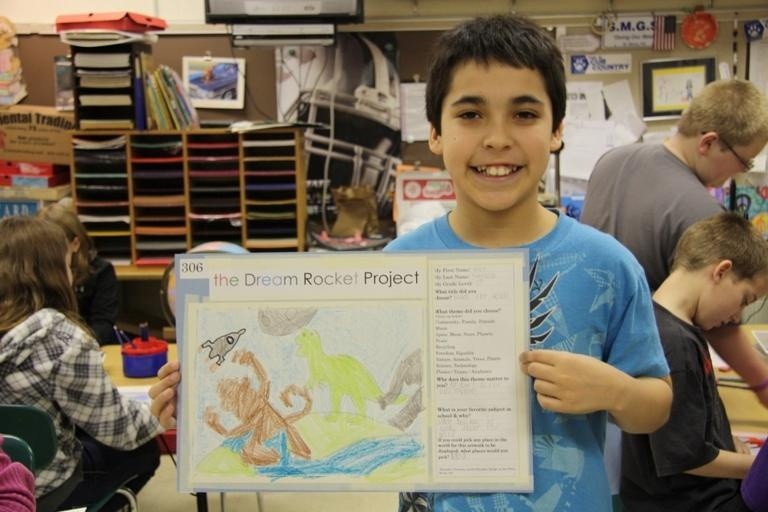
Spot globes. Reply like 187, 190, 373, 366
158, 240, 250, 335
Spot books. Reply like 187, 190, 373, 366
147, 65, 199, 132
79, 95, 132, 106
79, 119, 133, 129
76, 70, 134, 86
75, 53, 134, 68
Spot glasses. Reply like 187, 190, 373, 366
703, 130, 753, 173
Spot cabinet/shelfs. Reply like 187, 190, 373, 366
293, 75, 402, 245
64, 128, 310, 277
64, 38, 150, 129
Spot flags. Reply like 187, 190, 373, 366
654, 14, 676, 50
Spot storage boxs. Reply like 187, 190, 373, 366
4, 189, 64, 221
0, 104, 71, 164
0, 160, 69, 190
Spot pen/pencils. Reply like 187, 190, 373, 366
139, 322, 149, 341
120, 330, 136, 348
114, 325, 123, 346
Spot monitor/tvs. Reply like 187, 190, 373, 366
203, 0, 365, 26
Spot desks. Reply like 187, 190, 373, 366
99, 342, 207, 511
707, 322, 768, 458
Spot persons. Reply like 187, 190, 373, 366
36, 204, 121, 348
579, 79, 768, 411
148, 13, 672, 512
620, 214, 768, 512
0, 216, 166, 512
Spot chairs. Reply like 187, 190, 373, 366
0, 435, 34, 471
0, 407, 140, 512
